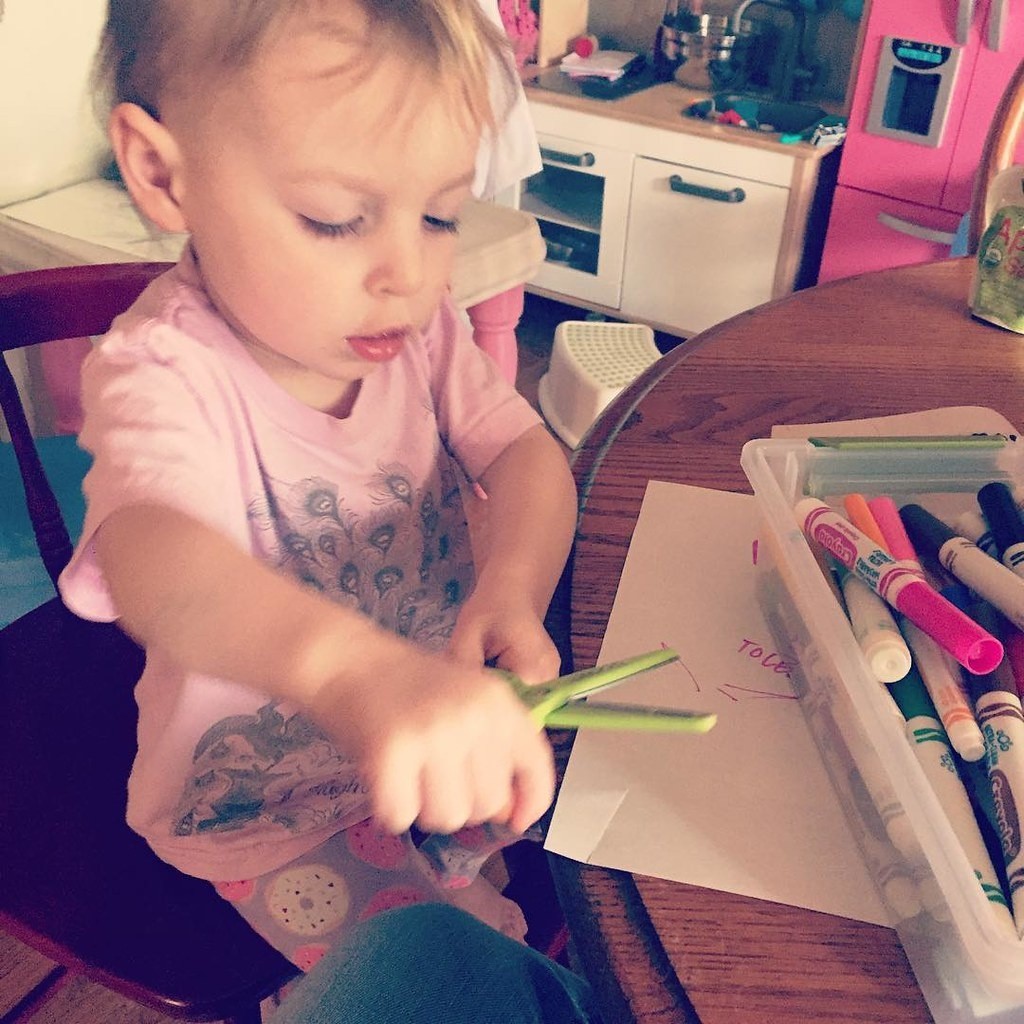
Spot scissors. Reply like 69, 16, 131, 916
490, 648, 718, 734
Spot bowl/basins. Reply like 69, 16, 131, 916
545, 233, 587, 261
661, 12, 765, 92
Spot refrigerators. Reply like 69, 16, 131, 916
818, 1, 1024, 287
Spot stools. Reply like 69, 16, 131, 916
537, 320, 667, 448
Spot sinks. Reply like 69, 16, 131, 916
684, 91, 828, 143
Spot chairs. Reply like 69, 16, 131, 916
0, 267, 564, 1024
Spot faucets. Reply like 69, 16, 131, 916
731, 0, 818, 100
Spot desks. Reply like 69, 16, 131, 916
543, 254, 1024, 1024
0, 176, 547, 437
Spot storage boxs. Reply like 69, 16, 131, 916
738, 429, 1024, 1023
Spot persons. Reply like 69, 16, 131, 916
56, 0, 578, 969
263, 904, 606, 1024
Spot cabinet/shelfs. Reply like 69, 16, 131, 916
484, 100, 824, 343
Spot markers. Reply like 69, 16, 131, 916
795, 474, 1024, 1016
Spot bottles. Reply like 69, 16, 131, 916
967, 163, 1024, 335
